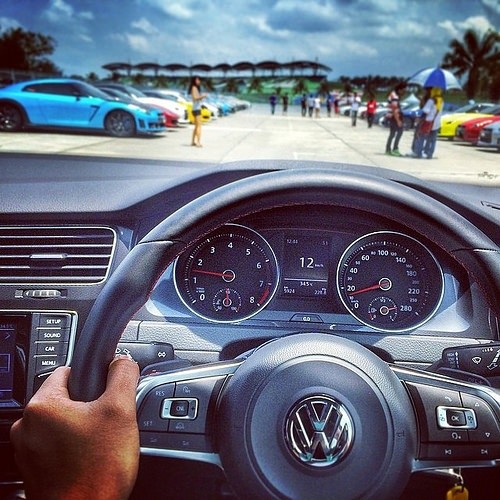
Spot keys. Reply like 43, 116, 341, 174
406, 468, 469, 500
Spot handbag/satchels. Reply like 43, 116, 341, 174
419, 120, 434, 136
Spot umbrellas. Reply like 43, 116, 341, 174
405, 67, 463, 97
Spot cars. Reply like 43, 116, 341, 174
338, 92, 500, 152
0, 78, 252, 137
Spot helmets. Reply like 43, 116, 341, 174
430, 87, 441, 98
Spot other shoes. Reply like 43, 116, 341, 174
392, 149, 402, 157
384, 149, 393, 156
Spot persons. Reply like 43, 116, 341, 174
188, 76, 207, 148
412, 87, 432, 158
350, 92, 361, 127
314, 95, 321, 118
301, 94, 307, 115
282, 92, 289, 116
415, 87, 444, 158
387, 82, 407, 157
309, 92, 314, 117
269, 92, 277, 114
9, 355, 140, 500
335, 90, 340, 113
368, 92, 377, 127
327, 95, 332, 117
